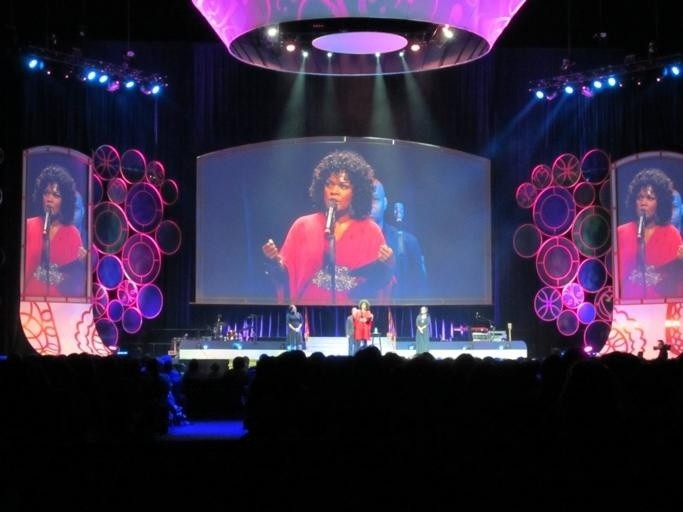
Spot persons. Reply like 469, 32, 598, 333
1, 347, 681, 511
285, 304, 304, 351
413, 305, 433, 355
615, 168, 683, 302
344, 306, 359, 356
73, 189, 87, 246
654, 339, 671, 360
352, 299, 373, 352
261, 150, 396, 307
669, 190, 683, 235
347, 178, 429, 299
25, 166, 88, 297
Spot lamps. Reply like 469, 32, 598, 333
192, 0, 526, 78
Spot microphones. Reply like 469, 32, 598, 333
324, 200, 336, 234
637, 210, 645, 237
42, 207, 51, 234
394, 203, 404, 224
